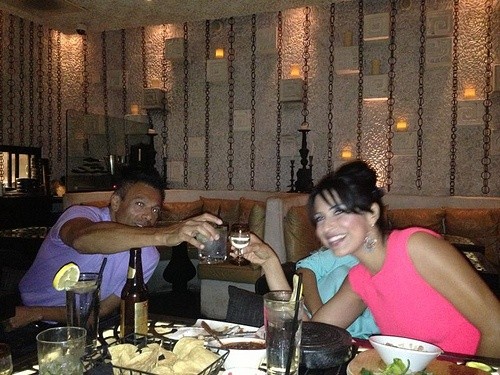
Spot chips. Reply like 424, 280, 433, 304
103, 336, 223, 375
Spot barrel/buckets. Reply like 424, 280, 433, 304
30, 157, 50, 187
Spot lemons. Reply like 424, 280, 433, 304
53, 263, 80, 291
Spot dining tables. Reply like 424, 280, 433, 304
0, 312, 500, 375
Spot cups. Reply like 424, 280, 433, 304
108, 154, 117, 175
0, 343, 13, 375
196, 226, 227, 264
263, 292, 304, 375
66, 272, 103, 351
36, 326, 87, 375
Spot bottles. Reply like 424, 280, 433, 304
120, 248, 149, 344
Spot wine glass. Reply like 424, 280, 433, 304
229, 224, 251, 267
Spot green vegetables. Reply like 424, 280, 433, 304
359, 358, 410, 375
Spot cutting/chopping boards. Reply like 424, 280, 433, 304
346, 349, 494, 375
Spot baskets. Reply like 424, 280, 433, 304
79, 332, 230, 375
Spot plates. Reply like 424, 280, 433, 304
220, 369, 268, 375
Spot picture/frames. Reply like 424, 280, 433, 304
139, 9, 500, 183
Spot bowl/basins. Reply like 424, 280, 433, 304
15, 178, 36, 192
266, 321, 359, 375
368, 335, 443, 373
207, 338, 266, 368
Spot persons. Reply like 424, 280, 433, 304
294, 242, 382, 339
232, 158, 500, 364
1, 171, 222, 359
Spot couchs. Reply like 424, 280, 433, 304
62, 187, 500, 321
